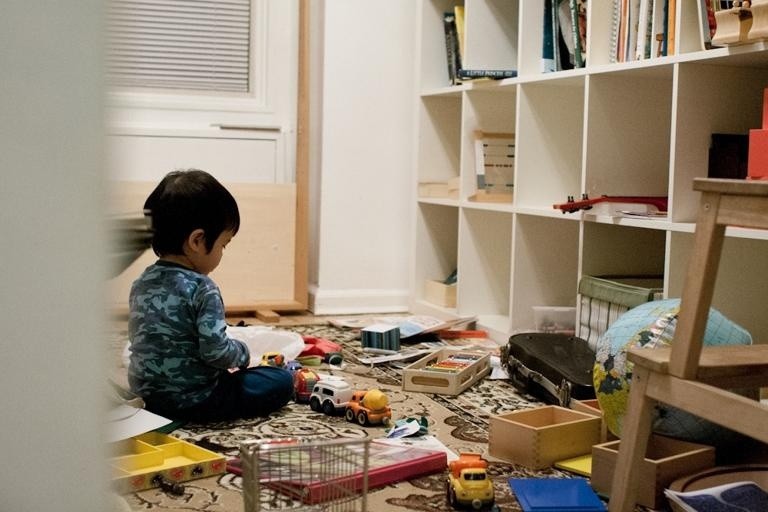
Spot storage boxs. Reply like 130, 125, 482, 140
422, 277, 457, 309
488, 398, 717, 512
576, 275, 663, 354
530, 305, 575, 333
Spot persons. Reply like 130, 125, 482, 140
128, 171, 292, 424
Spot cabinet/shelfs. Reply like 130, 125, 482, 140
407, 0, 766, 363
